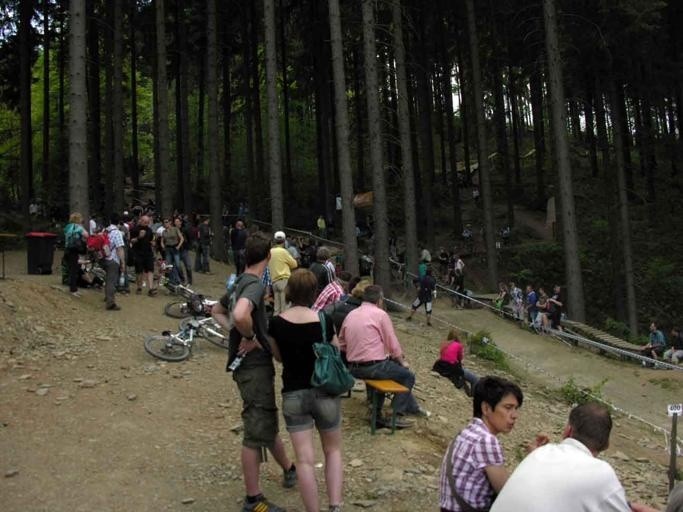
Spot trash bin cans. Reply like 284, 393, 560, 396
26, 231, 57, 275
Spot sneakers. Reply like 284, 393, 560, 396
242, 497, 286, 512
104, 297, 121, 310
136, 288, 158, 296
376, 408, 430, 429
284, 464, 296, 487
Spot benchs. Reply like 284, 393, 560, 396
469, 293, 683, 364
348, 379, 410, 435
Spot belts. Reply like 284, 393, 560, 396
359, 360, 380, 366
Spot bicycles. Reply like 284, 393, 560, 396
82, 258, 196, 297
145, 316, 229, 361
167, 294, 221, 335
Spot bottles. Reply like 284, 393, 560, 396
119, 273, 125, 290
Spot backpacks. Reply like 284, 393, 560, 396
87, 228, 116, 260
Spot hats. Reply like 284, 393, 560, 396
274, 231, 286, 240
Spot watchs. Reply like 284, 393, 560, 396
243, 333, 256, 341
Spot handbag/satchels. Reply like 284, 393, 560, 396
310, 342, 355, 396
68, 238, 86, 256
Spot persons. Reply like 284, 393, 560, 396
663, 327, 683, 365
437, 375, 551, 512
489, 401, 660, 512
268, 268, 342, 512
339, 285, 432, 428
223, 215, 565, 409
210, 230, 298, 512
640, 322, 666, 369
27, 198, 215, 311
